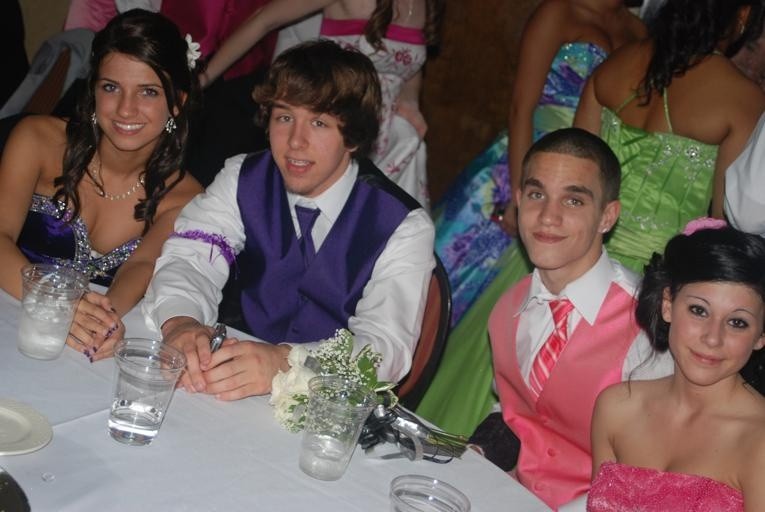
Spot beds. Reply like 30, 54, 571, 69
0, 278, 552, 511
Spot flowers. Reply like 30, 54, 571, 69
183, 32, 203, 71
271, 331, 398, 434
683, 216, 728, 236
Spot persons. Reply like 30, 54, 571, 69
585, 216, 763, 512
0, 9, 204, 363
63, 1, 430, 213
416, 0, 764, 438
146, 41, 437, 400
468, 125, 675, 512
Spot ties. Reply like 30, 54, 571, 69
293, 204, 321, 310
527, 298, 575, 405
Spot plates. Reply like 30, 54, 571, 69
0, 398, 54, 454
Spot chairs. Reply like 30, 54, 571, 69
389, 249, 453, 406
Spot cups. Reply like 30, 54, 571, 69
19, 261, 89, 361
298, 375, 378, 481
105, 338, 186, 447
388, 473, 471, 511
